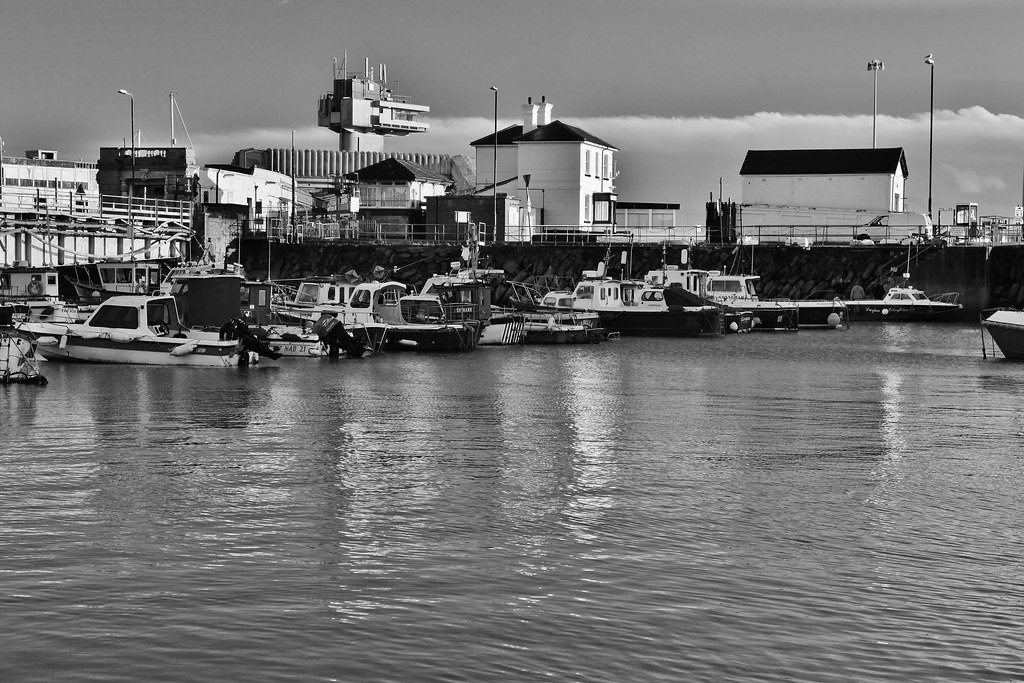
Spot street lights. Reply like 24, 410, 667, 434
118, 88, 136, 199
924, 57, 935, 219
867, 59, 885, 149
291, 128, 299, 229
489, 86, 498, 240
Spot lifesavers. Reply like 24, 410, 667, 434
136, 283, 147, 293
240, 282, 250, 296
28, 281, 43, 296
251, 273, 262, 282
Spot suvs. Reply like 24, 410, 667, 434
853, 211, 931, 242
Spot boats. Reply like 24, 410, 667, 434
2, 228, 1024, 369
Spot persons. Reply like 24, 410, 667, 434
850, 281, 866, 301
870, 278, 885, 300
